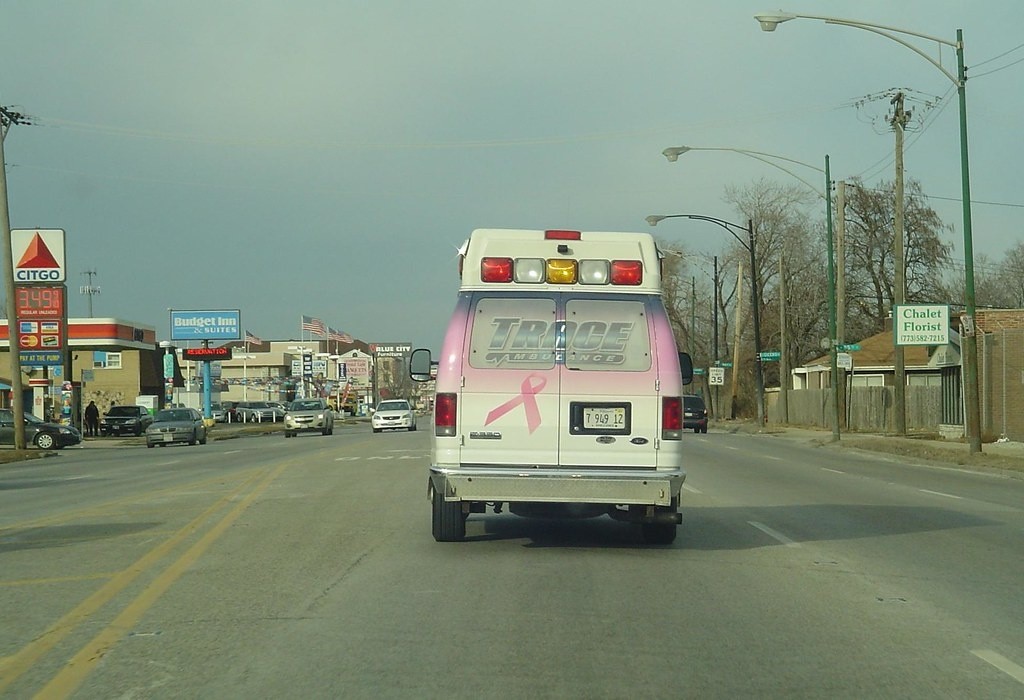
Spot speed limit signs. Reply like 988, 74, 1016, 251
709, 367, 724, 386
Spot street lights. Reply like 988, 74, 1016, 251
755, 14, 981, 452
644, 215, 765, 432
662, 146, 840, 441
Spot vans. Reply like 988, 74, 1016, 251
408, 229, 693, 545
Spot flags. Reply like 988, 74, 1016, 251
329, 327, 354, 344
303, 317, 326, 337
245, 329, 261, 346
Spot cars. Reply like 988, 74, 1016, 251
283, 398, 334, 438
683, 395, 708, 434
0, 408, 83, 450
371, 400, 416, 433
100, 406, 152, 437
211, 401, 288, 423
145, 408, 206, 448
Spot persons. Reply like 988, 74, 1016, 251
85, 401, 100, 437
